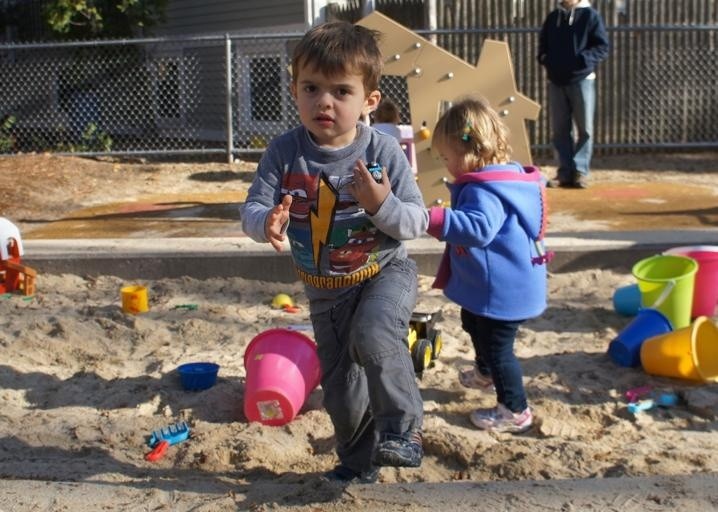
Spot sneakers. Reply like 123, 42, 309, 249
459, 365, 496, 394
573, 172, 587, 188
548, 177, 565, 187
317, 465, 378, 484
377, 429, 423, 468
470, 403, 532, 433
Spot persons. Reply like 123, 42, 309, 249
426, 99, 555, 434
536, 0, 609, 189
371, 102, 402, 143
239, 21, 429, 484
